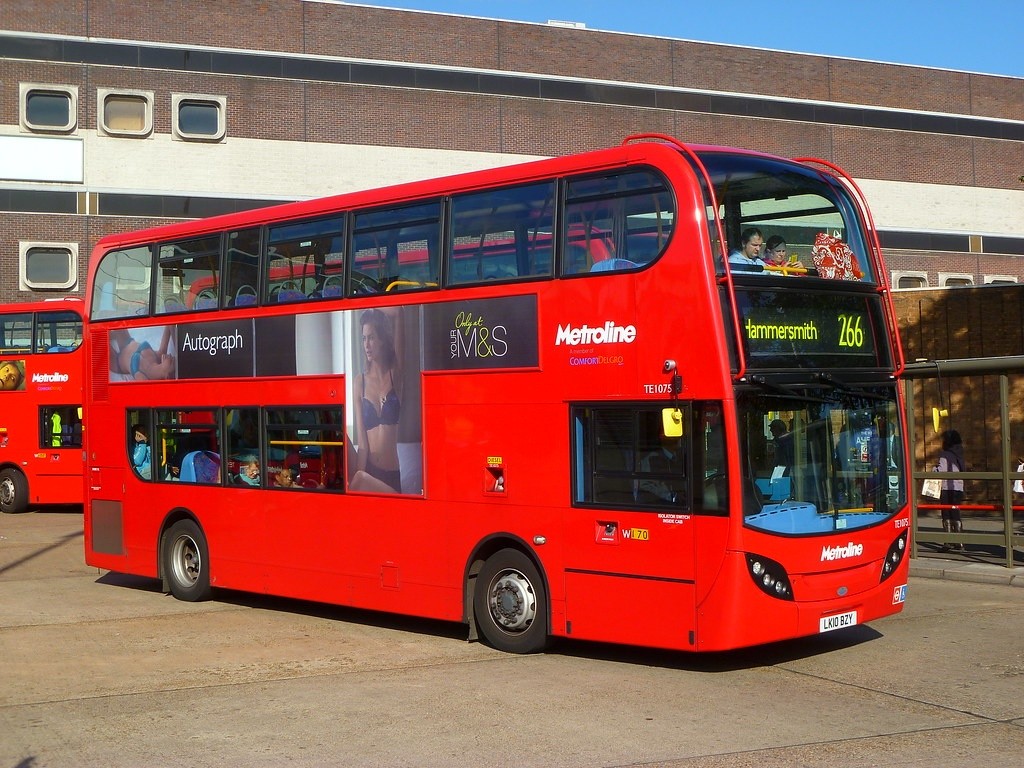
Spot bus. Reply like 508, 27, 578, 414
83, 131, 913, 661
0, 295, 85, 515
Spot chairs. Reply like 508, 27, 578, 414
589, 258, 638, 272
180, 450, 220, 483
195, 290, 217, 310
277, 280, 308, 302
235, 285, 257, 306
163, 294, 186, 312
321, 275, 342, 298
268, 285, 286, 302
47, 346, 76, 352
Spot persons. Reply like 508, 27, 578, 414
0, 361, 25, 390
234, 455, 261, 486
638, 427, 688, 505
109, 325, 177, 381
762, 234, 808, 276
932, 430, 970, 552
273, 465, 303, 488
769, 418, 807, 466
131, 424, 180, 480
347, 304, 406, 494
834, 416, 901, 498
727, 228, 768, 266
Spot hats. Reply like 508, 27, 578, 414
768, 420, 786, 430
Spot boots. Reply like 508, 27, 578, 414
937, 519, 952, 553
952, 521, 963, 549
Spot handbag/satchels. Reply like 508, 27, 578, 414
1012, 463, 1024, 493
921, 478, 942, 501
